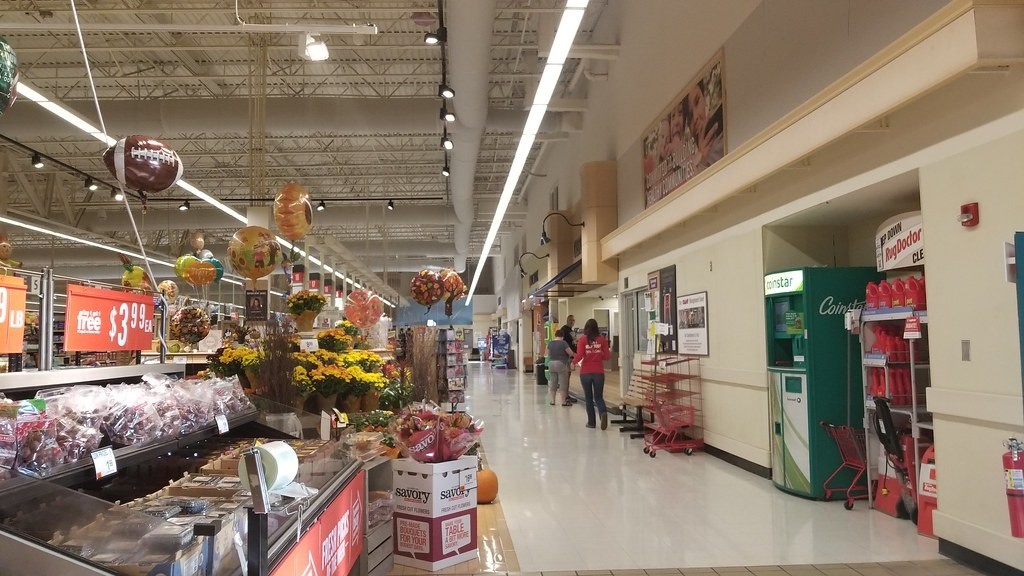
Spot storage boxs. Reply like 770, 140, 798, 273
392, 454, 478, 573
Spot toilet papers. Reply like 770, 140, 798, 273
238, 440, 300, 492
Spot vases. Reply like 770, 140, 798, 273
239, 373, 245, 388
295, 391, 309, 413
339, 393, 361, 412
245, 369, 262, 390
363, 385, 382, 411
317, 390, 337, 414
293, 310, 318, 332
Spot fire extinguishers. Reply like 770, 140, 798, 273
1002, 436, 1024, 538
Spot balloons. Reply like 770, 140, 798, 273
343, 289, 383, 330
439, 269, 463, 314
190, 230, 204, 250
170, 307, 211, 343
158, 279, 178, 302
102, 134, 183, 203
229, 225, 281, 281
0, 40, 18, 115
174, 249, 223, 285
122, 267, 151, 287
274, 184, 312, 241
410, 269, 444, 314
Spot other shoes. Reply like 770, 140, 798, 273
550, 401, 555, 405
601, 412, 607, 430
569, 397, 577, 402
586, 423, 595, 428
562, 401, 572, 406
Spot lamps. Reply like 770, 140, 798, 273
442, 166, 450, 176
519, 252, 550, 279
440, 108, 456, 122
424, 27, 447, 44
388, 200, 394, 211
85, 177, 98, 191
32, 153, 44, 169
297, 30, 330, 61
540, 213, 585, 245
317, 200, 325, 211
110, 188, 123, 201
440, 137, 453, 150
179, 201, 190, 211
438, 84, 455, 98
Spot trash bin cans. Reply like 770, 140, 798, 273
535, 357, 548, 385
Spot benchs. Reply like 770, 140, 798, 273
610, 371, 671, 440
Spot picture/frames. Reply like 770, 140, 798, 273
246, 290, 267, 321
641, 46, 728, 210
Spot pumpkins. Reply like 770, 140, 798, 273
475, 459, 498, 504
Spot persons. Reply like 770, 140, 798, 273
398, 328, 413, 358
252, 298, 261, 310
547, 330, 576, 406
560, 315, 577, 403
0, 242, 23, 277
645, 80, 722, 201
570, 319, 610, 429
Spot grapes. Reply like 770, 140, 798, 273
0, 389, 255, 483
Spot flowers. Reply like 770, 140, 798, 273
200, 289, 484, 462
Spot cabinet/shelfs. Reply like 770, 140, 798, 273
859, 306, 933, 509
24, 325, 136, 367
340, 349, 393, 360
440, 340, 467, 411
641, 352, 703, 458
360, 456, 394, 576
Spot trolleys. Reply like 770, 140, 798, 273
639, 356, 703, 458
819, 420, 880, 509
